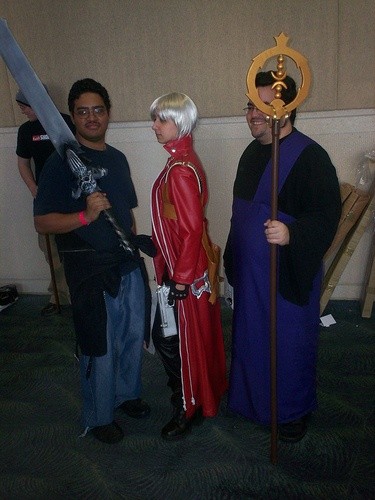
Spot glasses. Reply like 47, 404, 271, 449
73, 106, 107, 118
243, 104, 265, 115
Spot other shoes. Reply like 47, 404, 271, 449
41, 301, 71, 318
163, 394, 193, 440
279, 419, 307, 441
120, 396, 152, 418
85, 420, 126, 444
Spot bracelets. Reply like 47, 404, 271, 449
79, 211, 89, 226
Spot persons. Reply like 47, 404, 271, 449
149, 93, 228, 439
222, 72, 342, 443
33, 79, 150, 445
15, 81, 77, 317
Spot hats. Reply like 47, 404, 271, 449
15, 83, 48, 109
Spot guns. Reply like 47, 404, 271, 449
156, 282, 178, 338
